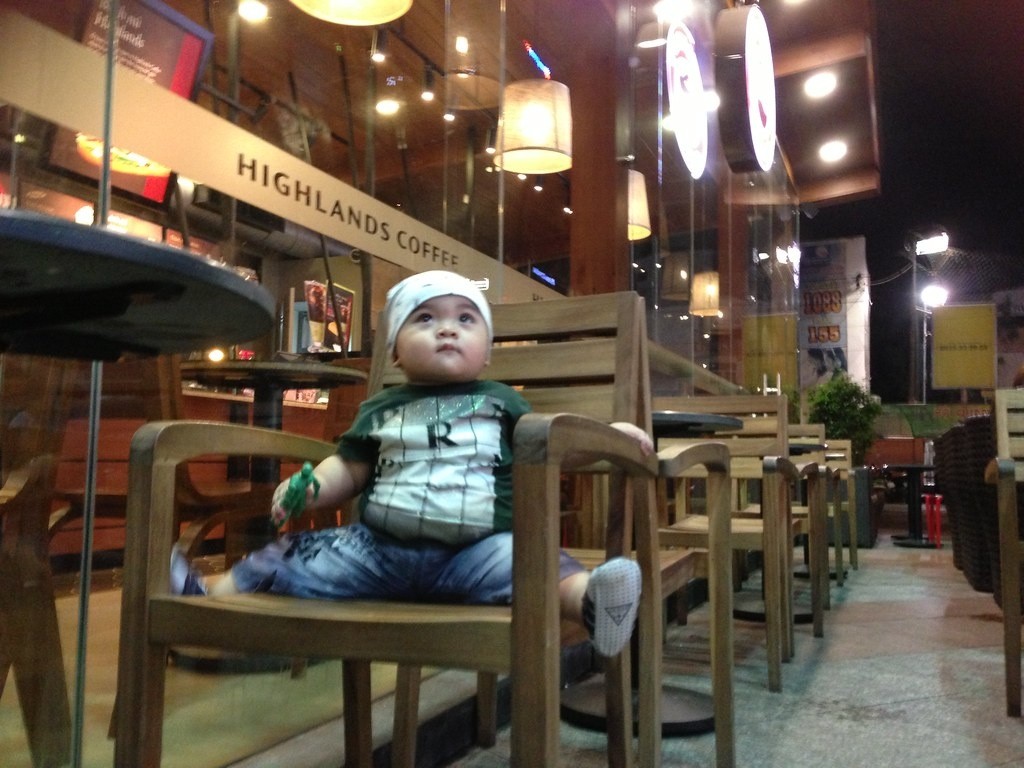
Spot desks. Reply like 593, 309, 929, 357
883, 464, 943, 549
0, 208, 277, 361
732, 443, 828, 624
560, 412, 743, 740
793, 454, 848, 579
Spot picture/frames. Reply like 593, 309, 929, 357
41, 0, 215, 215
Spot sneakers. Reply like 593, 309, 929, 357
581, 557, 642, 658
171, 542, 206, 595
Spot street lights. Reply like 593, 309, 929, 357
907, 225, 951, 402
918, 280, 949, 404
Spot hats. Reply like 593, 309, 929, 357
382, 269, 493, 359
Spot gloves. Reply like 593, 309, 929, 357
270, 459, 320, 531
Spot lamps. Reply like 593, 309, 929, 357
628, 169, 651, 241
493, 79, 572, 174
688, 272, 718, 317
445, 1, 505, 110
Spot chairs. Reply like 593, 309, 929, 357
984, 389, 1024, 717
1, 292, 858, 767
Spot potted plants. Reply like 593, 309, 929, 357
794, 369, 883, 551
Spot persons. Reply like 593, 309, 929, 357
988, 369, 1024, 455
806, 348, 847, 384
169, 270, 653, 656
271, 462, 321, 525
